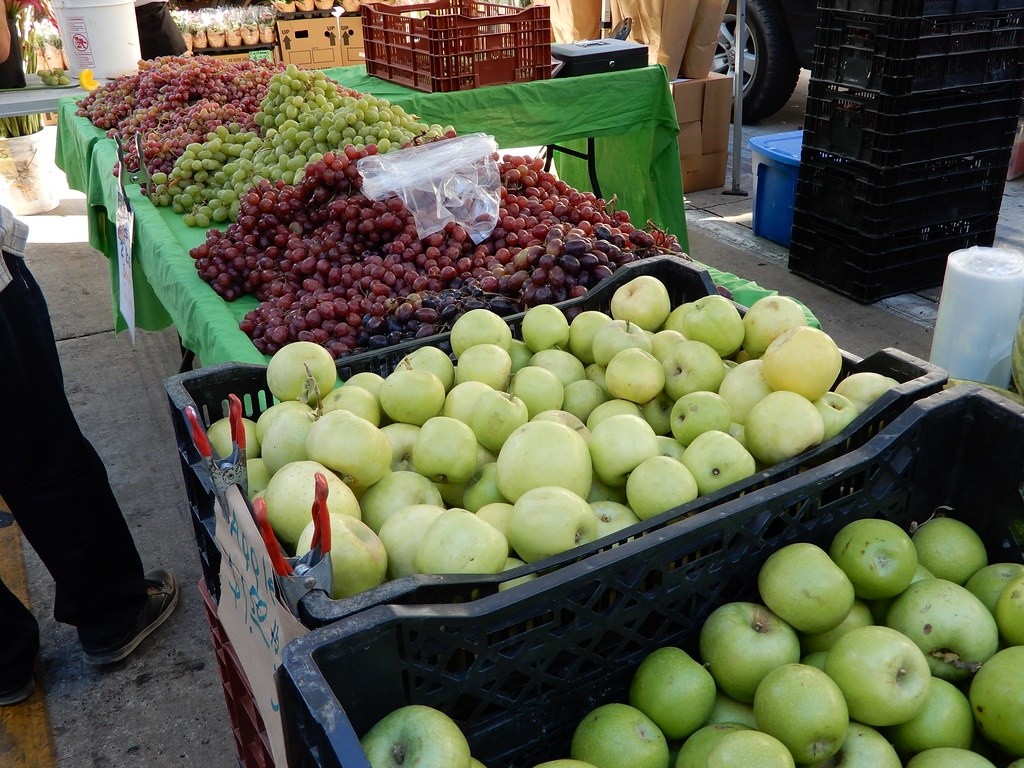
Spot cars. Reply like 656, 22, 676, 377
706, 0, 819, 126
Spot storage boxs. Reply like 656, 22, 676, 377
162, 255, 1024, 768
210, 50, 274, 65
747, 129, 803, 249
362, 0, 552, 93
551, 38, 649, 76
669, 71, 734, 194
787, 1, 1024, 306
277, 16, 367, 70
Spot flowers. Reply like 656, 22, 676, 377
0, 0, 59, 39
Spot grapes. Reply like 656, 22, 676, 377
190, 127, 732, 380
148, 62, 453, 227
75, 55, 366, 194
37, 67, 71, 86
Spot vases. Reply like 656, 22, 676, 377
0, 25, 26, 89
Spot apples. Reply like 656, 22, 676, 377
202, 274, 900, 599
359, 515, 1024, 768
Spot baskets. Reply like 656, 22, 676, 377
361, 0, 553, 94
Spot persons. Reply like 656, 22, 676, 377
0, 206, 178, 707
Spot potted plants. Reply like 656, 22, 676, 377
0, 30, 59, 215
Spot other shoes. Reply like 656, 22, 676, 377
76, 568, 179, 664
0, 619, 40, 706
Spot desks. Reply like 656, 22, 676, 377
0, 75, 111, 117
55, 62, 821, 415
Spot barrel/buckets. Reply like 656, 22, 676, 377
51, 1, 142, 79
0, 125, 60, 216
